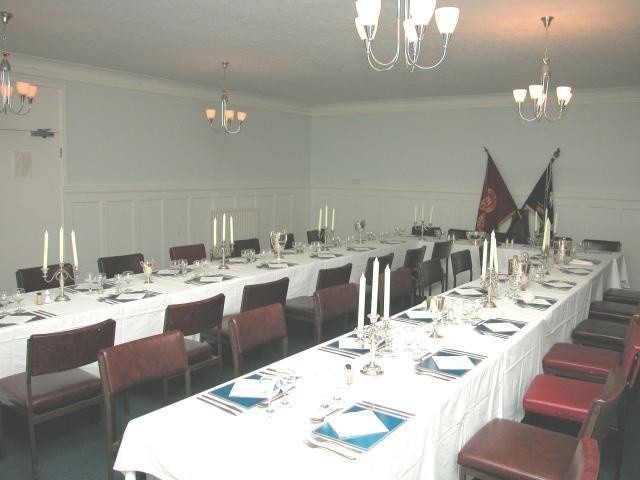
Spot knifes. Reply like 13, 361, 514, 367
357, 400, 415, 419
196, 394, 243, 415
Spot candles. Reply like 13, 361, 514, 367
534, 209, 558, 233
480, 229, 500, 280
354, 271, 368, 338
413, 204, 434, 224
41, 228, 79, 271
318, 205, 335, 231
540, 219, 552, 254
367, 254, 381, 326
382, 265, 393, 323
213, 214, 234, 247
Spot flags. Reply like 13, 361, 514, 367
526, 164, 554, 251
475, 152, 517, 232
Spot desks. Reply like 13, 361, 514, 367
0, 228, 440, 402
385, 227, 629, 296
118, 248, 614, 479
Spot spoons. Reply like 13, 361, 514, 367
309, 407, 343, 424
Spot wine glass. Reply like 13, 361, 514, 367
259, 371, 295, 414
0, 288, 25, 315
169, 258, 212, 279
240, 226, 484, 275
84, 271, 135, 297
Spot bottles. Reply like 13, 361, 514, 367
37, 290, 52, 304
345, 363, 354, 386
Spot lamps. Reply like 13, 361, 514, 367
355, 0, 459, 72
205, 63, 247, 134
512, 17, 572, 121
0, 11, 37, 114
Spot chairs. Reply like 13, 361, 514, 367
530, 235, 572, 248
540, 314, 637, 387
417, 257, 446, 301
271, 231, 297, 250
311, 283, 361, 343
586, 298, 636, 323
484, 231, 507, 243
150, 291, 226, 399
602, 284, 640, 305
167, 243, 208, 266
456, 362, 629, 479
378, 265, 416, 317
450, 248, 475, 287
96, 251, 146, 278
10, 260, 76, 291
0, 315, 118, 460
401, 245, 429, 268
227, 299, 290, 380
579, 237, 619, 253
199, 275, 291, 347
571, 316, 629, 350
285, 261, 354, 339
305, 229, 327, 244
446, 228, 479, 242
363, 250, 395, 283
229, 236, 261, 257
431, 239, 453, 277
410, 226, 443, 238
96, 328, 195, 478
562, 434, 603, 479
521, 322, 640, 476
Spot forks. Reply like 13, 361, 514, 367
414, 367, 457, 382
413, 351, 432, 363
301, 434, 362, 461
